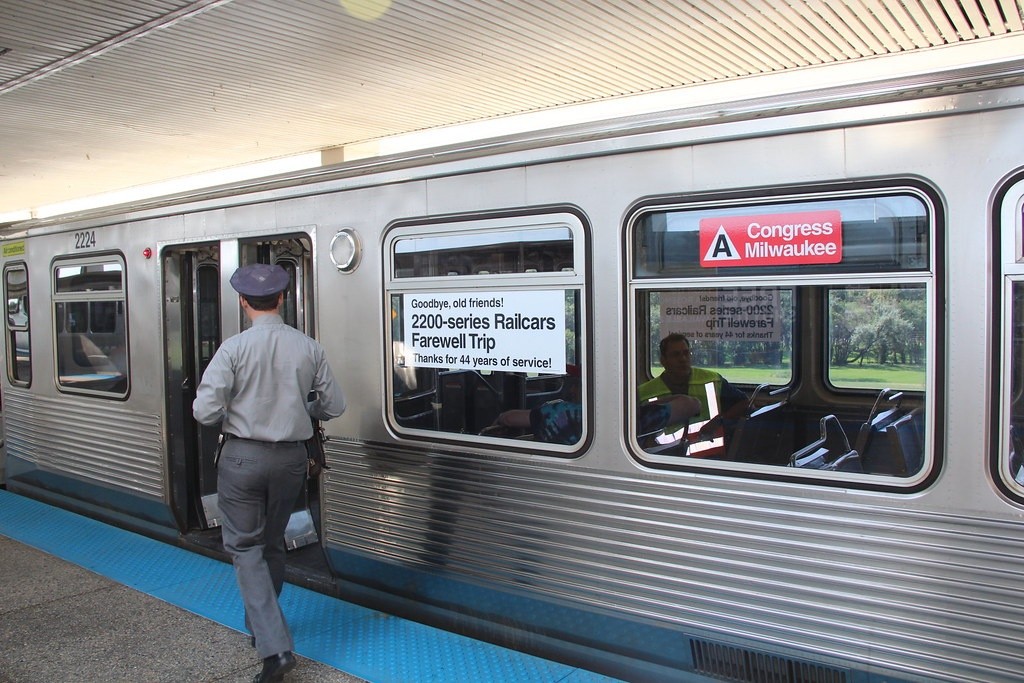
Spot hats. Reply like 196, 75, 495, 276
229, 263, 290, 298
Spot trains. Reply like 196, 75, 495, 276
0, 57, 1024, 683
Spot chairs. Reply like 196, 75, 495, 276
391, 365, 1023, 477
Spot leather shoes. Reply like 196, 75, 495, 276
252, 651, 297, 683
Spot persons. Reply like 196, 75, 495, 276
193, 264, 345, 683
637, 333, 755, 443
493, 381, 701, 446
